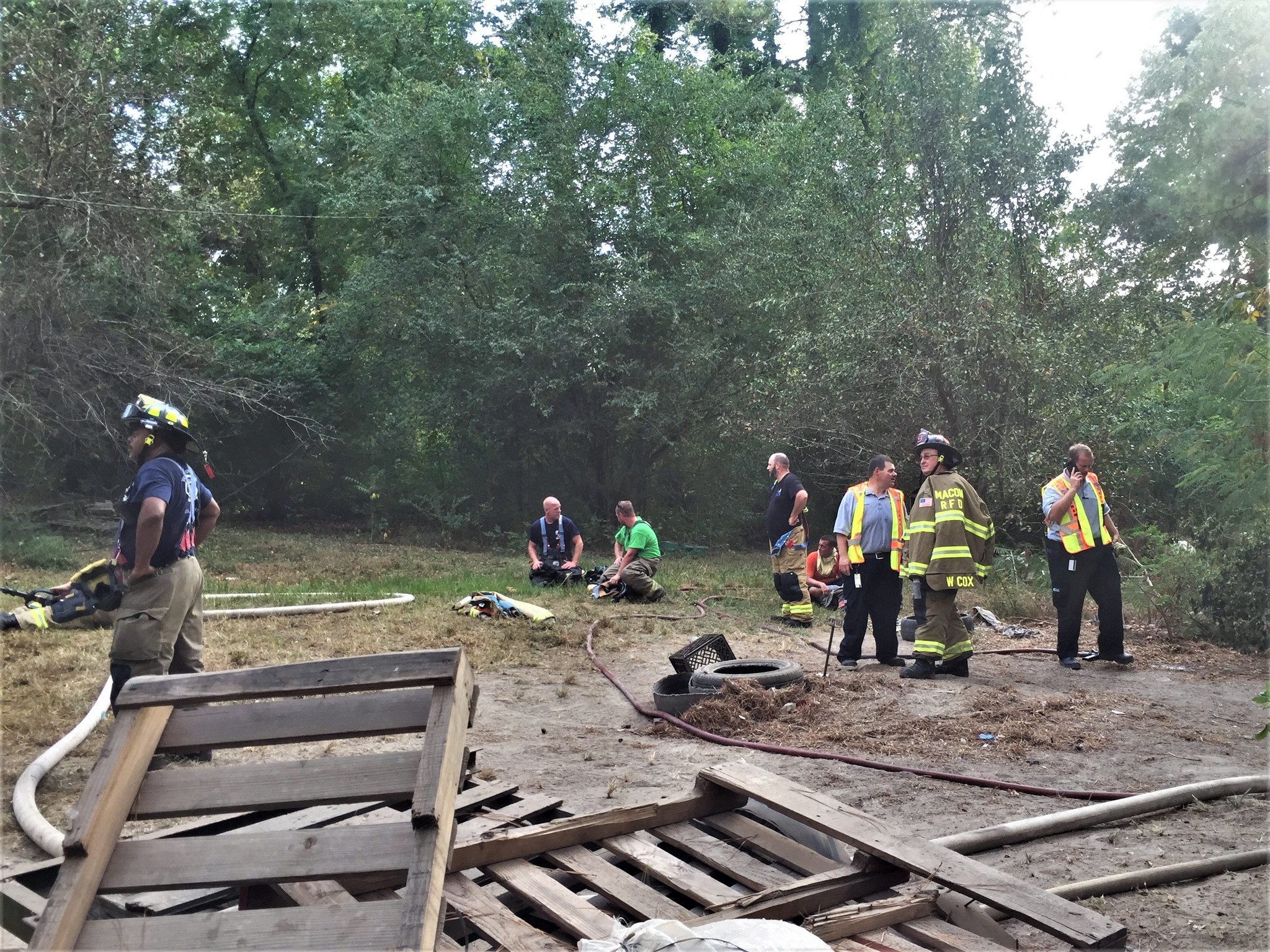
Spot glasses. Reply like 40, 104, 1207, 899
617, 500, 626, 514
917, 453, 938, 461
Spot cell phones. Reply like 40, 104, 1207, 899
1065, 459, 1079, 476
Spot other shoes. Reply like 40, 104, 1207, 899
784, 618, 812, 628
840, 658, 858, 670
882, 656, 906, 667
770, 616, 787, 623
1059, 652, 1081, 669
1099, 651, 1134, 664
935, 658, 969, 677
643, 588, 666, 604
899, 658, 936, 680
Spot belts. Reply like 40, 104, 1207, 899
864, 551, 891, 560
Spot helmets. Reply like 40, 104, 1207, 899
910, 428, 963, 469
120, 393, 207, 464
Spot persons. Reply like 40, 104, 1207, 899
899, 434, 973, 679
765, 452, 814, 628
833, 454, 907, 670
1040, 443, 1134, 670
806, 534, 847, 611
528, 497, 584, 588
0, 395, 222, 772
600, 500, 666, 604
